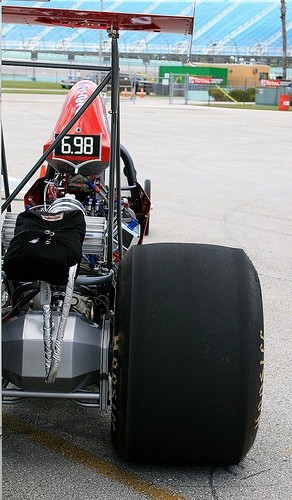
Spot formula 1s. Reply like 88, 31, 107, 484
2, 6, 266, 466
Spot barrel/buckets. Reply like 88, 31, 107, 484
277, 93, 291, 111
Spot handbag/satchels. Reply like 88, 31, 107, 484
3, 211, 85, 284
